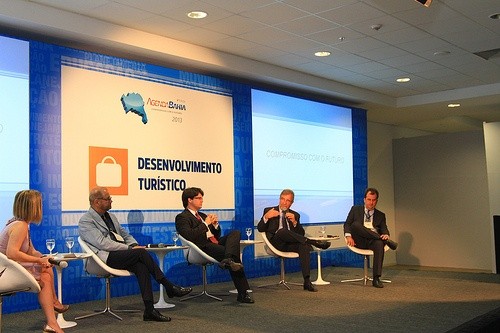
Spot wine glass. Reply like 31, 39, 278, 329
46, 239, 55, 255
65, 236, 75, 254
320, 226, 326, 237
172, 232, 179, 247
245, 228, 252, 241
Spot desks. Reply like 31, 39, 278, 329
306, 237, 340, 286
43, 253, 91, 329
144, 246, 189, 309
228, 240, 266, 294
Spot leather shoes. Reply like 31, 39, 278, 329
304, 282, 317, 292
387, 240, 397, 250
237, 293, 254, 303
372, 279, 383, 287
220, 259, 243, 271
315, 240, 331, 249
166, 287, 192, 298
143, 310, 171, 322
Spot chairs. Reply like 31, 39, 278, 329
0, 252, 41, 333
176, 233, 230, 302
74, 236, 141, 322
341, 238, 392, 285
258, 232, 304, 289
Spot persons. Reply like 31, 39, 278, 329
174, 187, 256, 304
0, 188, 71, 333
77, 187, 193, 322
257, 189, 331, 292
344, 188, 399, 288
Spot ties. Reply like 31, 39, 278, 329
195, 212, 218, 244
103, 215, 124, 242
367, 210, 370, 222
282, 210, 288, 231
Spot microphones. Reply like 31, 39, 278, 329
146, 243, 165, 248
48, 258, 68, 268
283, 207, 291, 222
324, 235, 339, 238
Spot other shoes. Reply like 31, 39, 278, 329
44, 324, 55, 333
54, 304, 70, 312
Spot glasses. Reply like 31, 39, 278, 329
96, 196, 110, 201
